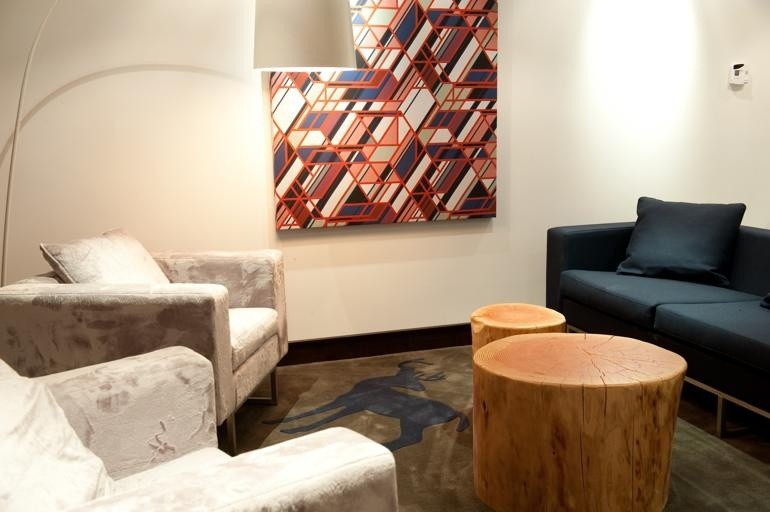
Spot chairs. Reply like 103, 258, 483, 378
0, 249, 290, 455
1, 346, 401, 511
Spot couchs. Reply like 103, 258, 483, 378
545, 220, 770, 449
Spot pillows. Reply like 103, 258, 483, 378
42, 226, 173, 289
616, 196, 748, 288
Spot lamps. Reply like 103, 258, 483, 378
253, 0, 357, 73
